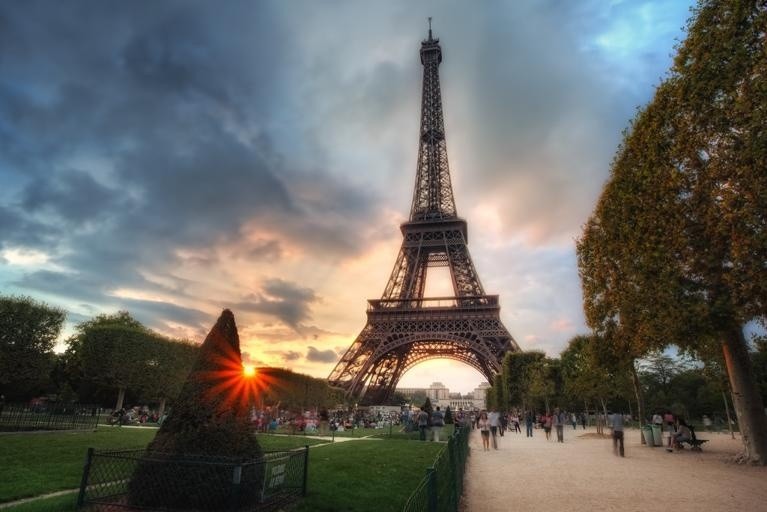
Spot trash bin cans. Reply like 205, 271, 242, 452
642, 425, 663, 447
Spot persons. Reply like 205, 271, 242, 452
702, 415, 712, 434
652, 410, 663, 433
610, 409, 625, 457
477, 411, 489, 452
664, 419, 691, 452
664, 410, 673, 425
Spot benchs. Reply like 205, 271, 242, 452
668, 434, 708, 450
